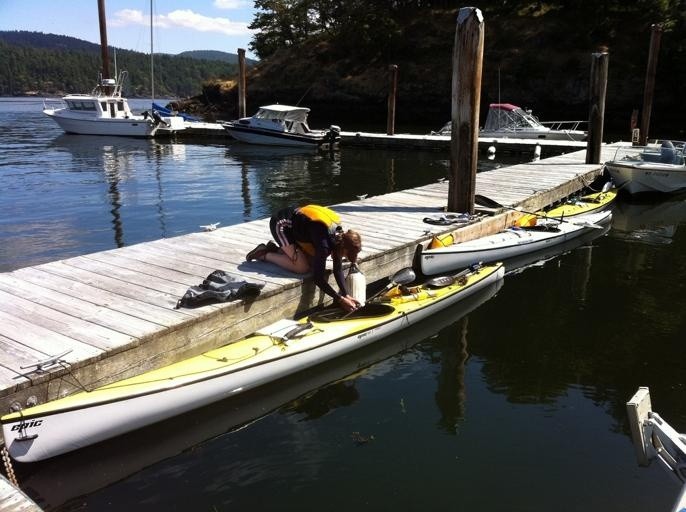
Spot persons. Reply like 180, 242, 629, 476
246, 204, 361, 313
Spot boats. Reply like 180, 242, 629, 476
604, 139, 686, 193
41, 78, 169, 137
221, 104, 342, 149
513, 188, 619, 228
0, 260, 505, 463
430, 101, 590, 141
419, 210, 613, 275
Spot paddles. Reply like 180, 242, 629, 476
342, 267, 416, 319
427, 264, 482, 286
474, 194, 604, 229
595, 182, 612, 201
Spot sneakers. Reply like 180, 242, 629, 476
246, 240, 276, 262
